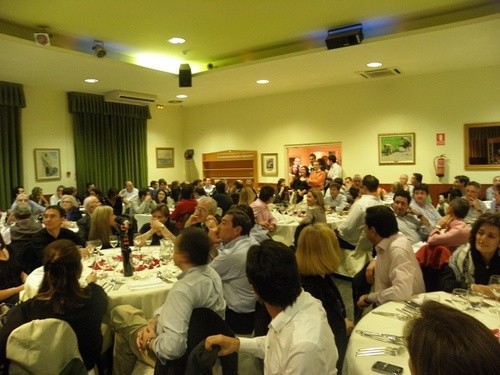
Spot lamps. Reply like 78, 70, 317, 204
92, 39, 109, 59
35, 24, 53, 48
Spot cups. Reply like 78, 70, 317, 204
270, 203, 344, 220
423, 288, 483, 310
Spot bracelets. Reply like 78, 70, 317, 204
435, 225, 442, 230
364, 294, 368, 302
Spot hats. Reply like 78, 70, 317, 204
412, 172, 422, 183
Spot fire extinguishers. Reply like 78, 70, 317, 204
434, 154, 447, 177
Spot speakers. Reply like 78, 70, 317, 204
184, 149, 194, 159
325, 28, 364, 50
179, 64, 192, 87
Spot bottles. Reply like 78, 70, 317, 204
38, 214, 42, 223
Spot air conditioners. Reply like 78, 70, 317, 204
105, 89, 158, 106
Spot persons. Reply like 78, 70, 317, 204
205, 239, 339, 375
0, 152, 500, 375
402, 300, 500, 375
0, 239, 109, 375
357, 205, 426, 306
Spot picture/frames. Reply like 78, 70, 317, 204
156, 146, 175, 168
34, 148, 62, 181
377, 133, 418, 165
261, 153, 279, 177
464, 121, 500, 172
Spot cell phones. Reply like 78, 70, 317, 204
372, 361, 403, 375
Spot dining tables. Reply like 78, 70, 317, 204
18, 245, 186, 360
340, 285, 500, 375
258, 204, 350, 246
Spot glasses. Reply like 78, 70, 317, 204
194, 207, 207, 214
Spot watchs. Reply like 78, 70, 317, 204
160, 224, 165, 229
146, 337, 156, 351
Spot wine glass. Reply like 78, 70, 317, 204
489, 275, 500, 307
75, 233, 173, 281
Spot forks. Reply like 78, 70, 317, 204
371, 307, 422, 321
355, 347, 406, 357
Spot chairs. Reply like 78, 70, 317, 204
133, 305, 240, 375
5, 318, 86, 375
